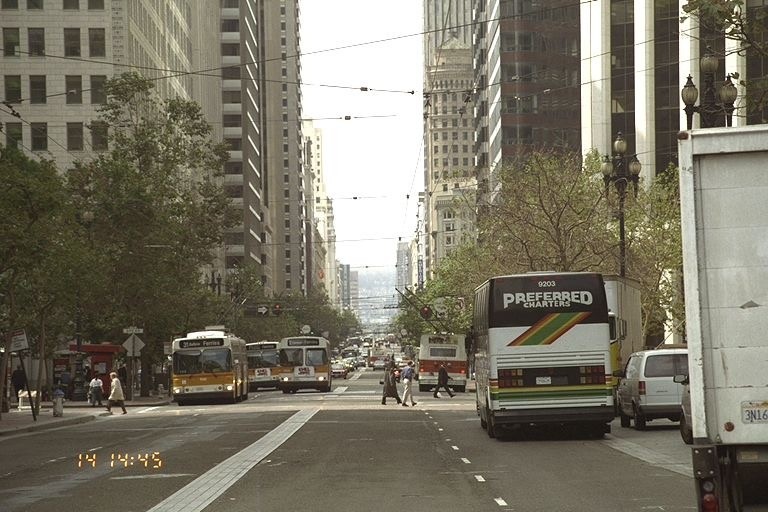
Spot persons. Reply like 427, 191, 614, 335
89, 373, 104, 407
11, 364, 27, 403
401, 360, 418, 407
106, 372, 127, 415
433, 361, 456, 398
118, 362, 128, 393
60, 364, 91, 400
381, 360, 402, 405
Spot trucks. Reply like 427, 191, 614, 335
600, 273, 644, 389
676, 124, 768, 511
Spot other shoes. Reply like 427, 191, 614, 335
402, 403, 409, 407
412, 402, 417, 406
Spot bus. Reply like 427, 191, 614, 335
277, 335, 333, 393
166, 325, 249, 407
418, 332, 468, 393
245, 340, 281, 391
464, 270, 617, 440
368, 347, 394, 366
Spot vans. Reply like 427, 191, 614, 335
347, 335, 373, 345
616, 348, 689, 430
674, 374, 693, 444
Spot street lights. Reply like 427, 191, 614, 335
601, 131, 643, 277
680, 43, 738, 129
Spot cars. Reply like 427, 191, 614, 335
373, 360, 386, 371
390, 343, 416, 371
328, 343, 371, 380
